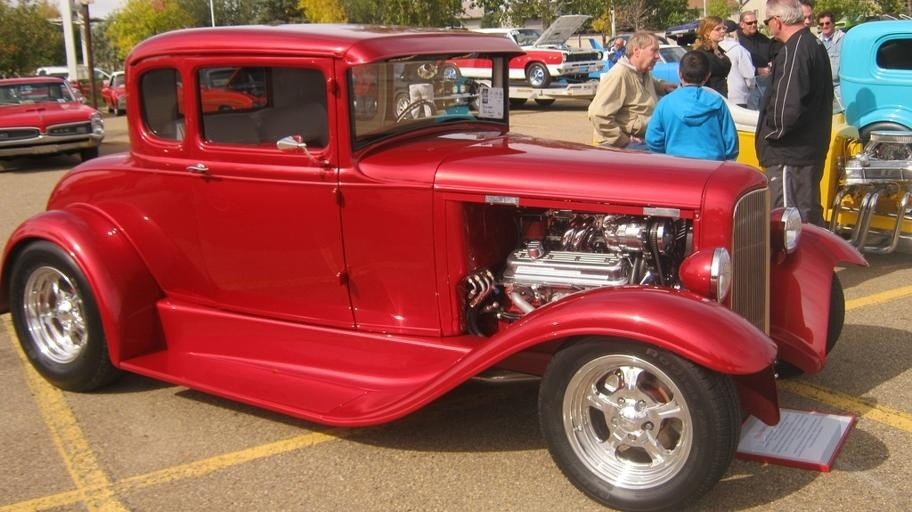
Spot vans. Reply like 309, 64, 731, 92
834, 18, 912, 159
36, 65, 107, 83
206, 69, 258, 95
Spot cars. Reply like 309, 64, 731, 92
0, 76, 107, 162
72, 79, 104, 98
609, 31, 670, 50
441, 15, 607, 87
100, 69, 127, 115
586, 44, 691, 89
173, 84, 264, 113
666, 21, 705, 44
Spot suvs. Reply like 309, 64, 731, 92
0, 24, 871, 510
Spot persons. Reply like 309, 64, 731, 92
586, 27, 682, 151
690, 0, 847, 113
752, 0, 835, 232
644, 49, 740, 162
353, 61, 379, 120
607, 36, 627, 69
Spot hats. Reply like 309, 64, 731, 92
726, 20, 738, 34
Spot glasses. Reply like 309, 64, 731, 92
764, 16, 779, 26
819, 22, 830, 26
746, 21, 757, 25
712, 26, 728, 31
